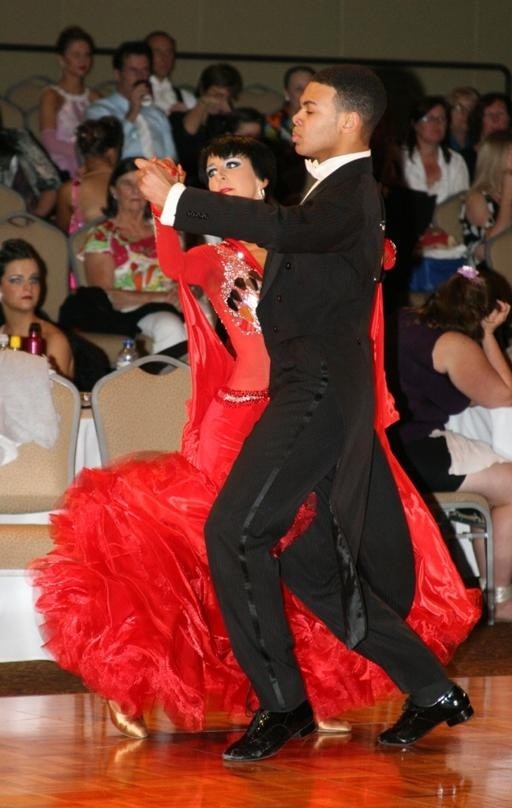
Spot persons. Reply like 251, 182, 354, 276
33, 132, 483, 739
133, 61, 475, 763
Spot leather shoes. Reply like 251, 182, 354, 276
375, 682, 477, 750
218, 695, 320, 763
100, 685, 150, 744
309, 707, 356, 736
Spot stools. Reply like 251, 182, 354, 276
431, 490, 498, 628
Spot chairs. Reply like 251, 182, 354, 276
411, 179, 511, 323
89, 354, 192, 471
0, 347, 82, 572
2, 75, 111, 327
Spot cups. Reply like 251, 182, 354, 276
135, 80, 156, 109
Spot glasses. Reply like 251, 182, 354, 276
417, 101, 477, 124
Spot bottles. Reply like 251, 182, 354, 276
115, 338, 139, 368
26, 322, 46, 356
1, 333, 22, 351
